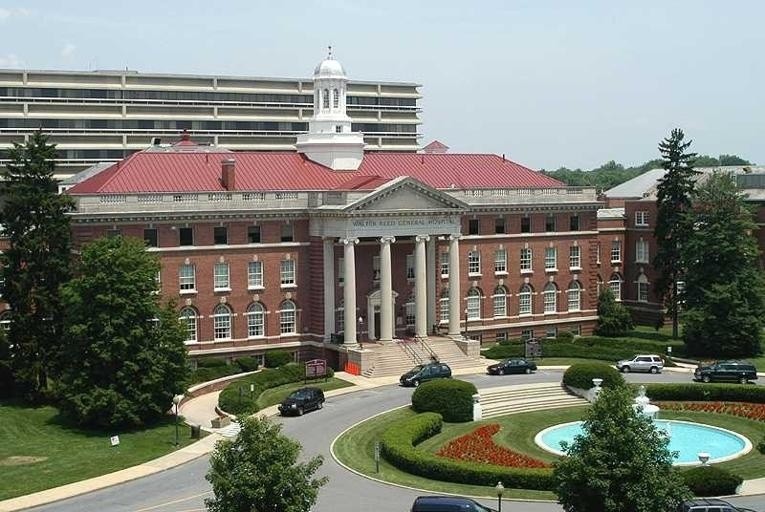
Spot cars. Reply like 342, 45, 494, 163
487, 359, 536, 375
694, 362, 757, 384
411, 496, 504, 512
616, 355, 665, 374
678, 499, 756, 512
400, 363, 451, 386
278, 387, 325, 415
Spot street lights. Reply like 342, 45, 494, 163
496, 481, 504, 512
464, 308, 468, 335
358, 317, 363, 347
173, 396, 179, 444
